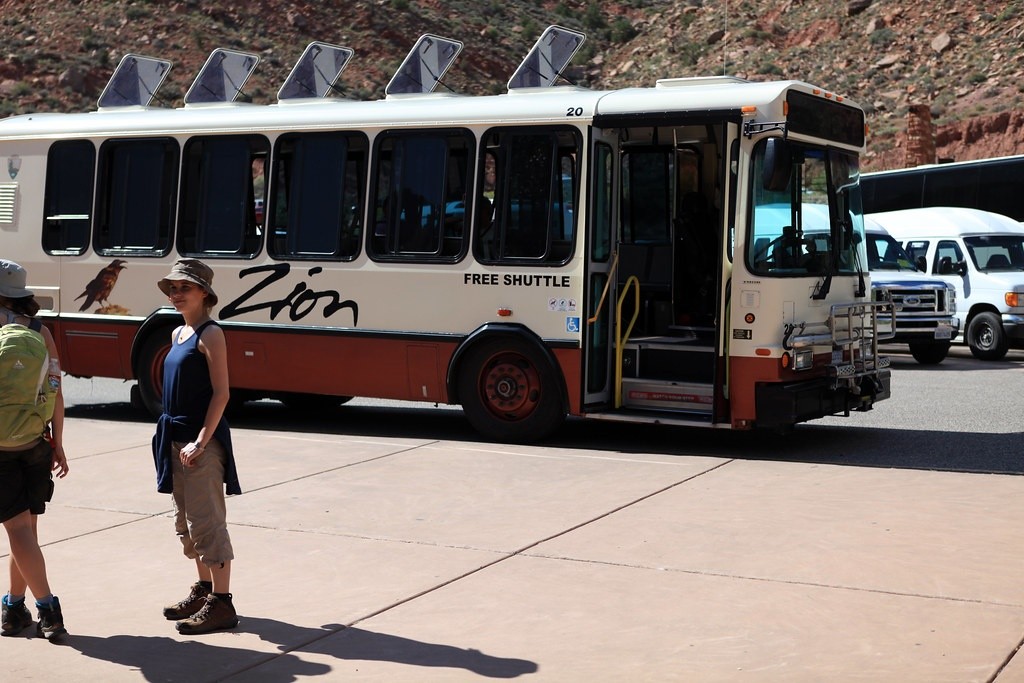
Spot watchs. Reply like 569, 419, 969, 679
194, 440, 205, 454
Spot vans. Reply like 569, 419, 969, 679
752, 201, 1024, 363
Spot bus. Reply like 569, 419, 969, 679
860, 155, 1024, 270
0, 22, 896, 446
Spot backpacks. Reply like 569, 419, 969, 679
0, 318, 56, 448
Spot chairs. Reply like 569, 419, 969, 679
937, 256, 952, 274
987, 254, 1010, 268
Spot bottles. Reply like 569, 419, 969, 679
48, 358, 60, 392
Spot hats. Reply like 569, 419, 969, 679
157, 258, 218, 308
0, 259, 34, 297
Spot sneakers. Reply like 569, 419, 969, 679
0, 595, 33, 636
176, 594, 238, 634
35, 596, 67, 640
164, 583, 211, 620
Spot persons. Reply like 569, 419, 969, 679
0, 260, 69, 641
152, 258, 243, 635
